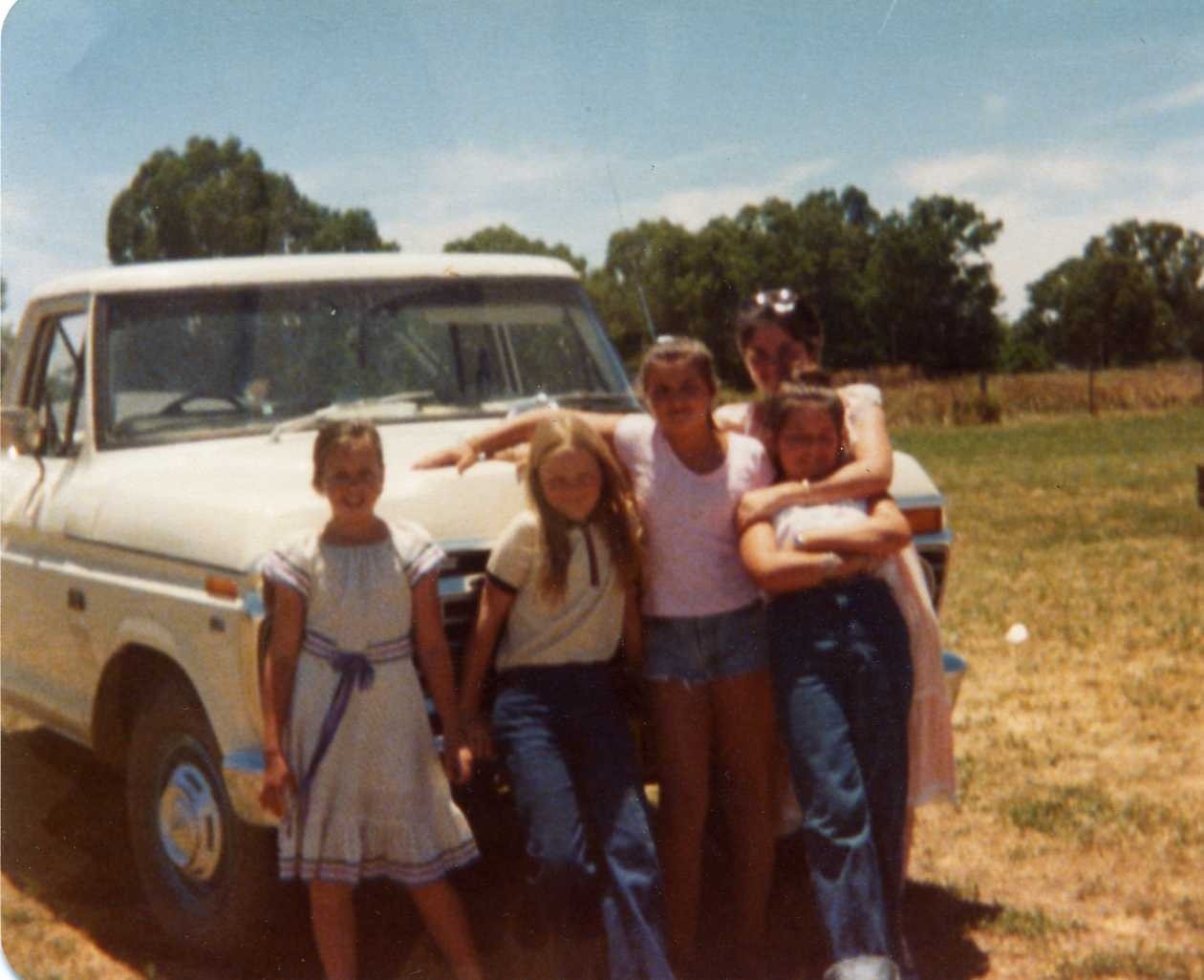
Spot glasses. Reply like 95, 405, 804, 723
752, 288, 799, 306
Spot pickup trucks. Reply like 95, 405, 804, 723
0, 247, 950, 951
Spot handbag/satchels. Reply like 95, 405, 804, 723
771, 736, 802, 837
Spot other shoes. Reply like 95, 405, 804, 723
735, 932, 768, 977
669, 948, 699, 979
888, 915, 919, 978
526, 863, 566, 935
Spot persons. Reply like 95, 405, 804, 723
260, 418, 480, 980
439, 283, 951, 980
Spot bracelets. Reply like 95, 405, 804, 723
799, 477, 813, 509
